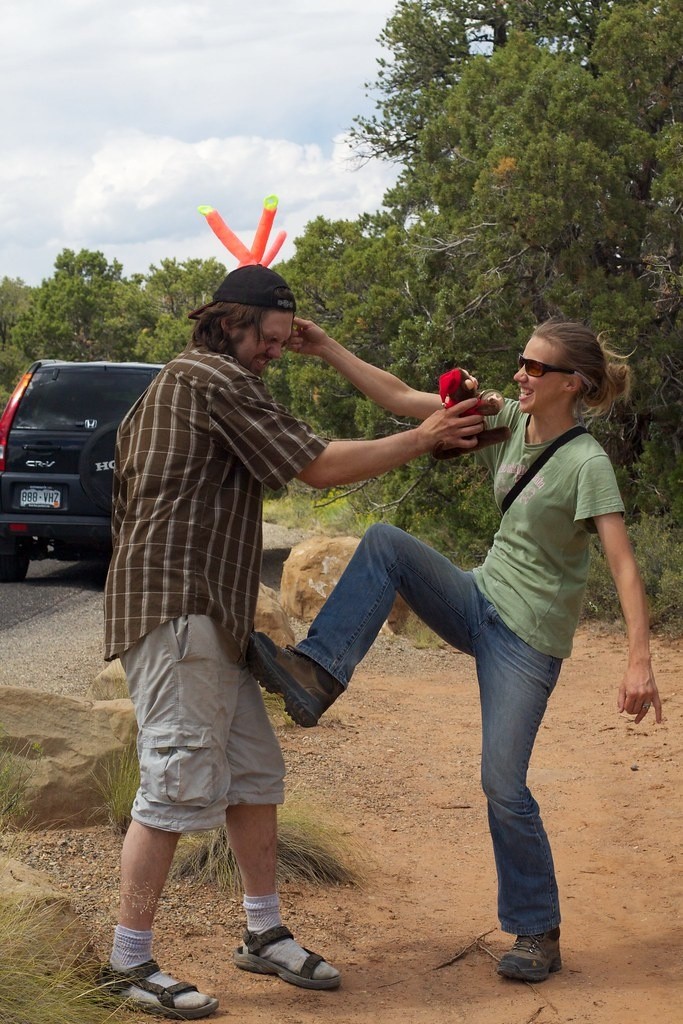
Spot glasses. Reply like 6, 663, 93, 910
517, 353, 593, 390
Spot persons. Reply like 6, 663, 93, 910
249, 317, 662, 980
106, 266, 497, 1018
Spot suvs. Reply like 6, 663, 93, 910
0, 360, 170, 582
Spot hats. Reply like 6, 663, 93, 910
188, 263, 295, 321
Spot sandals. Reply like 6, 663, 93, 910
234, 927, 340, 990
100, 961, 218, 1020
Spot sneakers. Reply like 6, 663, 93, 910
248, 632, 343, 727
497, 928, 561, 981
